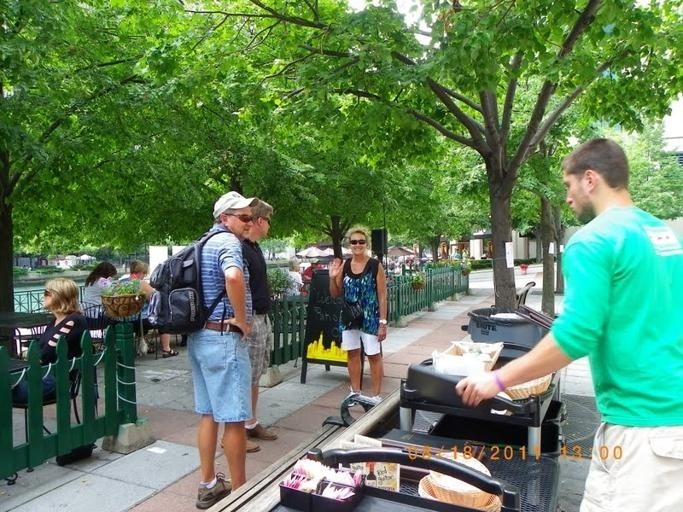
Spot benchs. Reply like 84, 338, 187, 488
204, 387, 401, 512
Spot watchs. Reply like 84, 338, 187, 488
378, 319, 387, 325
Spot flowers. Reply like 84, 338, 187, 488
97, 277, 145, 297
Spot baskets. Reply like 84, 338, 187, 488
506, 373, 552, 399
418, 451, 502, 512
435, 341, 503, 376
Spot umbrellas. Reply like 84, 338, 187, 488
388, 245, 415, 256
297, 247, 351, 258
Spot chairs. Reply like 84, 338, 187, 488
25, 373, 82, 472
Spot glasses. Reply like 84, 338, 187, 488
349, 239, 366, 245
227, 214, 253, 222
44, 289, 53, 296
261, 216, 271, 225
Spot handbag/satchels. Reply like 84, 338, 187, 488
341, 301, 365, 327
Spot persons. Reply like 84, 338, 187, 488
119, 260, 178, 358
456, 137, 683, 511
186, 190, 259, 509
12, 278, 92, 403
327, 227, 387, 406
281, 258, 305, 297
303, 257, 324, 281
84, 262, 118, 339
221, 198, 279, 453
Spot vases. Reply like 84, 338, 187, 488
101, 294, 146, 316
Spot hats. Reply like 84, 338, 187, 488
212, 191, 259, 218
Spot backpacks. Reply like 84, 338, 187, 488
148, 231, 235, 336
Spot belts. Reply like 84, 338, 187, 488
205, 320, 240, 332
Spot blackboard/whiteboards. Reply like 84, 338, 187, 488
306, 273, 348, 363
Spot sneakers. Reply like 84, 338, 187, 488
343, 392, 362, 406
221, 437, 259, 453
244, 423, 277, 441
197, 471, 232, 509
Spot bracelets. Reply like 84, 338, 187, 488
492, 369, 506, 391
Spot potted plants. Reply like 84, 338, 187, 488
462, 266, 471, 275
411, 272, 425, 289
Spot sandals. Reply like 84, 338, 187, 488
162, 348, 179, 357
147, 347, 156, 353
